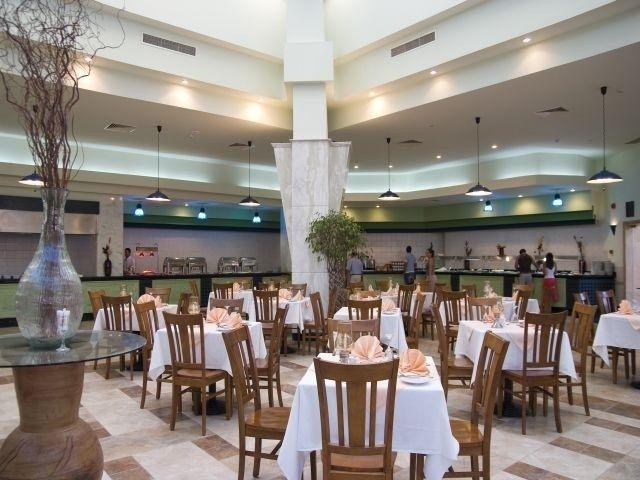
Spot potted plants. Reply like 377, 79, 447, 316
306, 208, 373, 310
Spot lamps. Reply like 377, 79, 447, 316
146, 126, 172, 201
377, 138, 400, 201
484, 193, 562, 211
18, 105, 49, 187
586, 85, 623, 184
610, 224, 617, 235
238, 141, 261, 207
465, 117, 494, 196
134, 204, 262, 223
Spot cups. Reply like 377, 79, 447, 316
188, 297, 202, 314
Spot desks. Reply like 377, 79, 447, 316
0, 329, 149, 480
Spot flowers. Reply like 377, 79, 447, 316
102, 237, 112, 258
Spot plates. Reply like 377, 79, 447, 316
217, 321, 251, 333
484, 319, 542, 331
400, 374, 431, 384
376, 308, 399, 314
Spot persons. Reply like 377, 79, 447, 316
515, 249, 540, 299
425, 249, 437, 292
404, 245, 416, 284
345, 251, 365, 283
123, 248, 136, 276
543, 253, 559, 314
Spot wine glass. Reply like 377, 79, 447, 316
55, 310, 69, 352
334, 319, 353, 366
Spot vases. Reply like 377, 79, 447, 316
104, 256, 112, 276
579, 260, 586, 273
15, 186, 84, 351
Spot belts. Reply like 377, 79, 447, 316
350, 274, 362, 275
